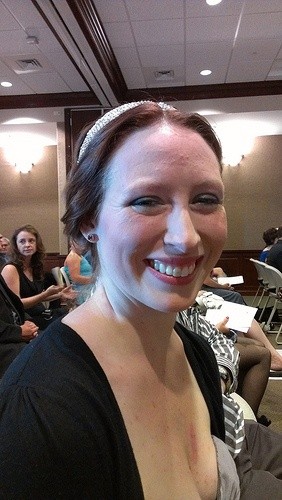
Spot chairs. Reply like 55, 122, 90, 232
60, 266, 76, 313
250, 258, 282, 345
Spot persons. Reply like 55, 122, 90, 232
0, 89, 282, 500
